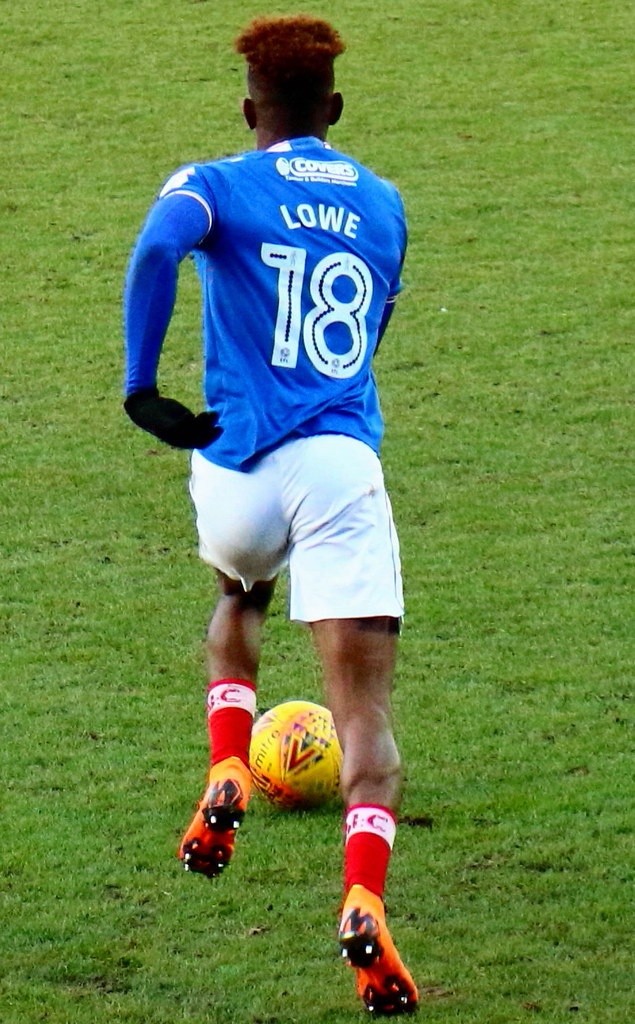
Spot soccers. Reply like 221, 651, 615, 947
247, 700, 345, 813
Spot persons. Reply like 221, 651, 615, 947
123, 20, 418, 1017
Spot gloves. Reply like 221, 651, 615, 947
123, 386, 223, 450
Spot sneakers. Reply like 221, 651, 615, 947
177, 756, 252, 878
337, 884, 419, 1020
